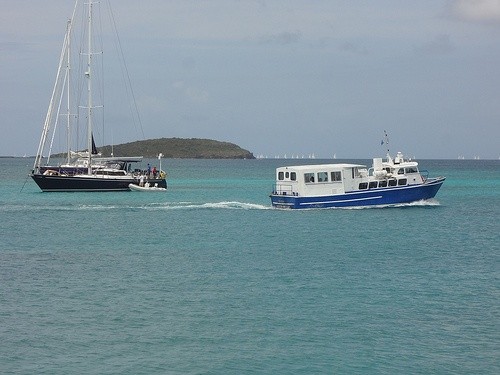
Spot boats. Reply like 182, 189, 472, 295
128, 151, 168, 193
270, 129, 447, 210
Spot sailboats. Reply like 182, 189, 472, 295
19, 1, 168, 193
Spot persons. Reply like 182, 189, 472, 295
135, 164, 165, 179
